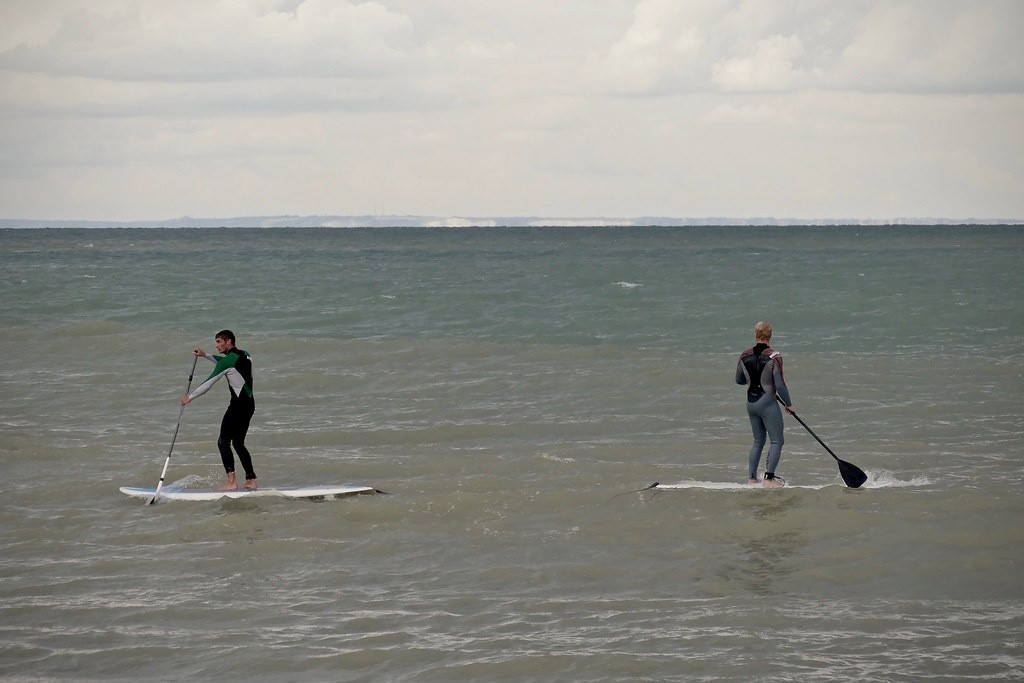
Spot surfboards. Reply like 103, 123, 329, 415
656, 480, 893, 490
119, 486, 373, 501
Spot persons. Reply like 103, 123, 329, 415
735, 322, 795, 488
181, 329, 258, 491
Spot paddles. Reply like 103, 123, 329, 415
147, 354, 199, 505
776, 395, 867, 488
